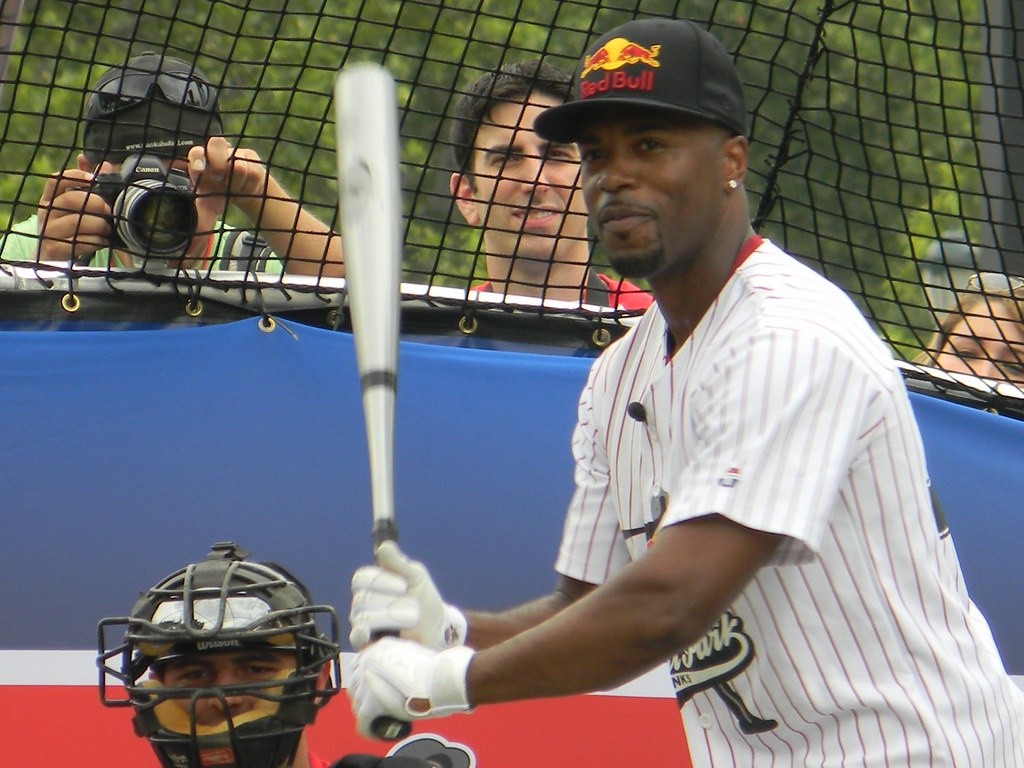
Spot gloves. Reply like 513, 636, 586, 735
347, 636, 476, 737
348, 540, 468, 650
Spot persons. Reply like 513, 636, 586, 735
349, 20, 1024, 768
449, 58, 653, 313
96, 539, 442, 768
0, 51, 345, 279
914, 271, 1024, 391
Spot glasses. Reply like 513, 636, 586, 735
962, 275, 1023, 297
82, 69, 225, 152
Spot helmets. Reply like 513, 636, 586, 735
97, 542, 341, 768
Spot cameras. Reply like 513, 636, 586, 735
65, 154, 198, 261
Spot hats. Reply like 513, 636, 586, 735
533, 18, 746, 145
81, 52, 222, 165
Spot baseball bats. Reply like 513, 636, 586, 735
332, 57, 413, 742
